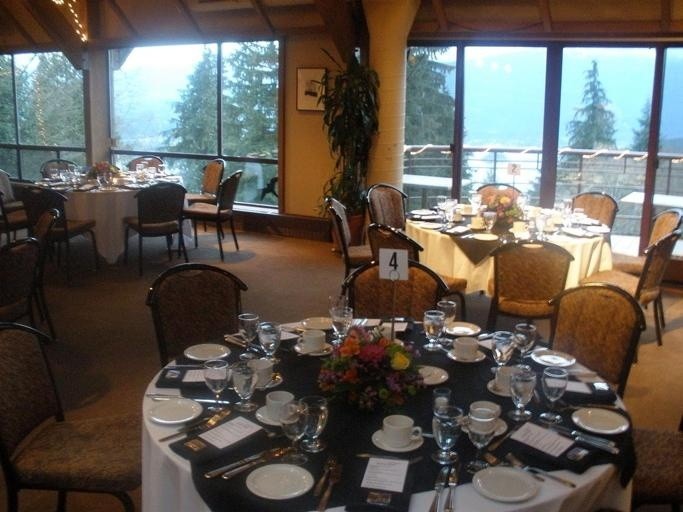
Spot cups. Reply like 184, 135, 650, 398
463, 205, 472, 213
470, 217, 483, 228
451, 336, 478, 359
266, 392, 297, 421
79, 174, 87, 186
512, 221, 529, 232
432, 389, 451, 416
296, 330, 325, 352
496, 367, 511, 390
382, 414, 422, 448
246, 359, 273, 385
470, 401, 501, 425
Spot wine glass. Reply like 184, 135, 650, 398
422, 309, 445, 352
135, 164, 143, 180
437, 196, 446, 217
297, 395, 328, 453
147, 167, 156, 183
483, 212, 497, 234
471, 194, 481, 214
158, 165, 165, 179
277, 402, 307, 465
103, 173, 114, 191
444, 209, 454, 228
492, 331, 515, 370
430, 406, 464, 465
437, 300, 456, 345
202, 359, 230, 412
328, 307, 353, 346
515, 323, 537, 370
257, 320, 281, 363
532, 199, 584, 243
538, 366, 568, 424
509, 364, 536, 422
467, 410, 496, 474
49, 169, 57, 183
231, 367, 258, 412
96, 175, 105, 191
68, 164, 75, 179
59, 171, 67, 185
236, 313, 260, 360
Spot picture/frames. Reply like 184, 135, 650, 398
295, 66, 328, 112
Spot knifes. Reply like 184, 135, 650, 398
203, 449, 281, 479
546, 426, 619, 455
541, 419, 616, 446
222, 448, 289, 479
223, 334, 260, 349
443, 467, 458, 511
428, 466, 448, 511
144, 393, 193, 400
225, 337, 259, 353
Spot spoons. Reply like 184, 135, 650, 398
316, 463, 342, 511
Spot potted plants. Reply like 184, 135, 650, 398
314, 41, 382, 255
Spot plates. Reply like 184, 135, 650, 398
466, 224, 486, 230
420, 222, 442, 229
460, 211, 476, 216
370, 429, 424, 452
166, 176, 179, 181
485, 379, 513, 397
570, 407, 630, 435
294, 342, 332, 356
444, 321, 481, 337
417, 365, 449, 386
585, 225, 608, 233
183, 344, 231, 361
411, 209, 431, 216
300, 317, 333, 330
274, 323, 303, 341
471, 466, 539, 504
509, 228, 529, 235
446, 348, 486, 363
380, 322, 407, 333
253, 375, 282, 388
531, 350, 576, 367
245, 463, 315, 501
472, 233, 498, 241
125, 184, 140, 189
254, 406, 300, 426
459, 415, 506, 436
146, 399, 203, 425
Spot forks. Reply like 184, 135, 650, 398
505, 452, 576, 488
314, 455, 337, 496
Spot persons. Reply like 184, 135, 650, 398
0, 168, 15, 247
